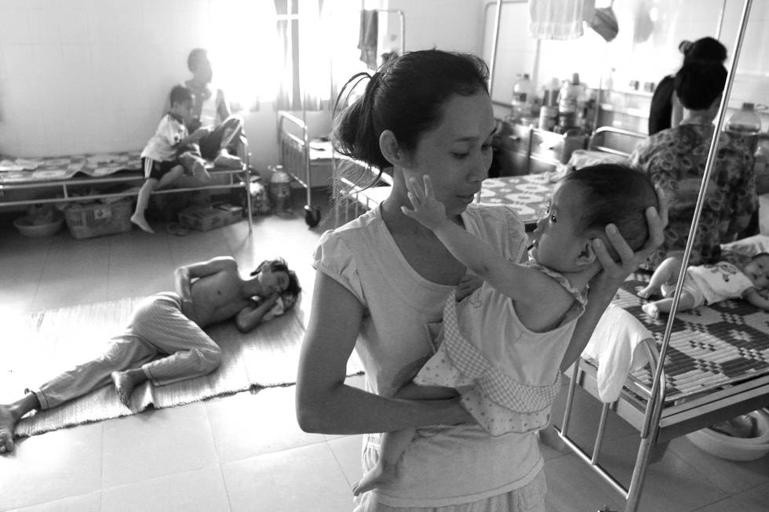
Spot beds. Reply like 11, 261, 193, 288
550, 233, 769, 512
0, 135, 254, 235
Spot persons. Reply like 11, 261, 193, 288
648, 36, 728, 137
636, 251, 768, 319
291, 47, 667, 512
162, 47, 245, 183
350, 163, 662, 497
626, 59, 765, 276
131, 85, 212, 234
0, 253, 302, 454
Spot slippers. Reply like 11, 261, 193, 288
166, 222, 177, 235
176, 223, 186, 235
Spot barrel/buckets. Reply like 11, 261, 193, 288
266, 164, 293, 217
508, 72, 536, 125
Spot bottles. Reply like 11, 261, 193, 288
511, 73, 585, 135
270, 165, 292, 215
725, 102, 761, 157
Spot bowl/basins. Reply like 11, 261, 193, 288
674, 384, 769, 462
13, 216, 64, 240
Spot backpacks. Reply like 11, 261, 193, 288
233, 165, 272, 216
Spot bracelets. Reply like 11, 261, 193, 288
182, 298, 194, 304
